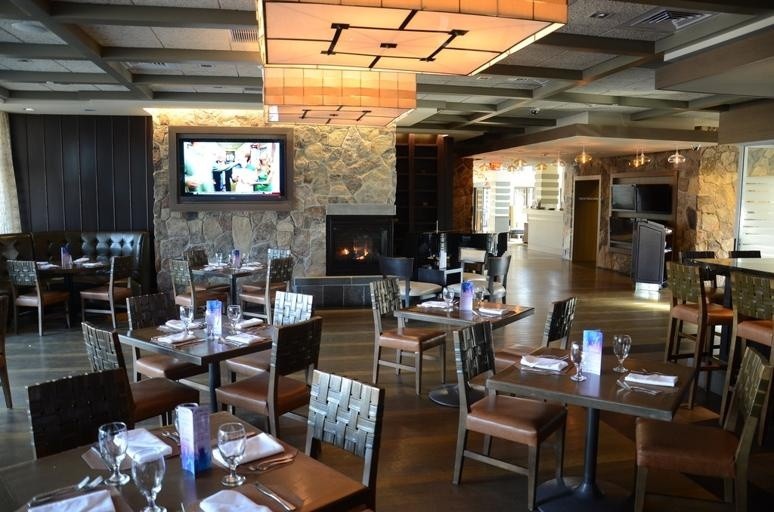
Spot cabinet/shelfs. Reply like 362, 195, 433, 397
604, 169, 682, 256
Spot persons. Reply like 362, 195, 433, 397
184, 141, 275, 193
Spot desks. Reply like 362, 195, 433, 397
0, 411, 372, 512
487, 346, 697, 512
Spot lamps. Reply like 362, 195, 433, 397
260, 67, 416, 129
477, 147, 690, 177
255, 1, 567, 77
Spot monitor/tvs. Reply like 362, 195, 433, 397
178, 137, 285, 201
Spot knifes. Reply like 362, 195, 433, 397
253, 480, 295, 511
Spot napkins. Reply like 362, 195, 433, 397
114, 428, 173, 465
211, 433, 284, 470
624, 373, 679, 387
198, 489, 272, 512
27, 489, 116, 512
520, 354, 569, 372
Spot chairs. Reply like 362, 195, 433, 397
0, 216, 149, 339
453, 320, 569, 510
494, 297, 577, 406
371, 227, 535, 396
305, 368, 385, 512
83, 248, 322, 445
630, 347, 773, 512
667, 250, 773, 445
24, 367, 136, 460
0, 291, 13, 409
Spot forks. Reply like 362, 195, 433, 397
615, 379, 663, 397
21, 474, 103, 508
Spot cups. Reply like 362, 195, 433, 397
175, 403, 198, 435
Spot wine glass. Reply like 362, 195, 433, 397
613, 335, 630, 372
227, 304, 240, 334
97, 422, 130, 485
215, 252, 248, 268
216, 421, 245, 487
131, 447, 166, 511
441, 286, 453, 307
179, 305, 193, 334
569, 340, 587, 382
